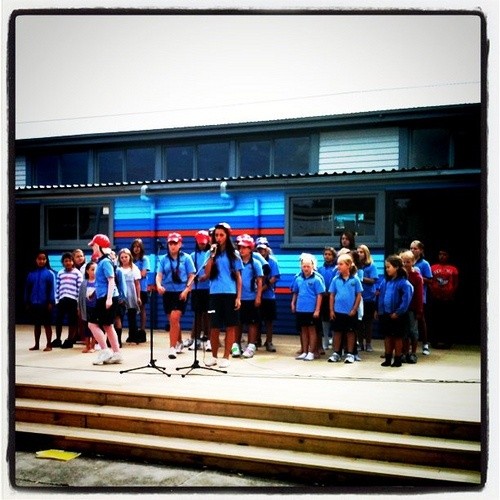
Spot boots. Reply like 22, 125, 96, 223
391, 355, 402, 367
381, 352, 393, 366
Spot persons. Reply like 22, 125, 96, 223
427, 248, 458, 349
291, 230, 379, 363
155, 233, 197, 358
190, 228, 281, 358
87, 234, 124, 365
375, 240, 433, 367
202, 223, 244, 368
23, 250, 57, 351
46, 238, 151, 352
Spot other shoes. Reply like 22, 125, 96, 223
365, 343, 372, 351
205, 357, 217, 366
51, 339, 73, 348
219, 358, 230, 368
422, 344, 430, 355
126, 330, 146, 342
401, 353, 418, 363
355, 355, 361, 360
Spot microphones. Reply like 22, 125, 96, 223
210, 243, 218, 251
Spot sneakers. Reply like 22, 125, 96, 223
344, 353, 355, 363
92, 348, 124, 365
206, 339, 212, 351
168, 340, 183, 359
265, 341, 276, 352
328, 352, 341, 362
231, 342, 241, 357
296, 352, 314, 361
183, 338, 201, 350
243, 342, 257, 358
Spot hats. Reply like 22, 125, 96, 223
257, 244, 267, 249
195, 230, 211, 241
88, 234, 110, 247
167, 232, 182, 242
237, 234, 253, 250
119, 248, 131, 254
255, 236, 269, 245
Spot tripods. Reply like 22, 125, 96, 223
119, 246, 172, 376
176, 252, 226, 377
